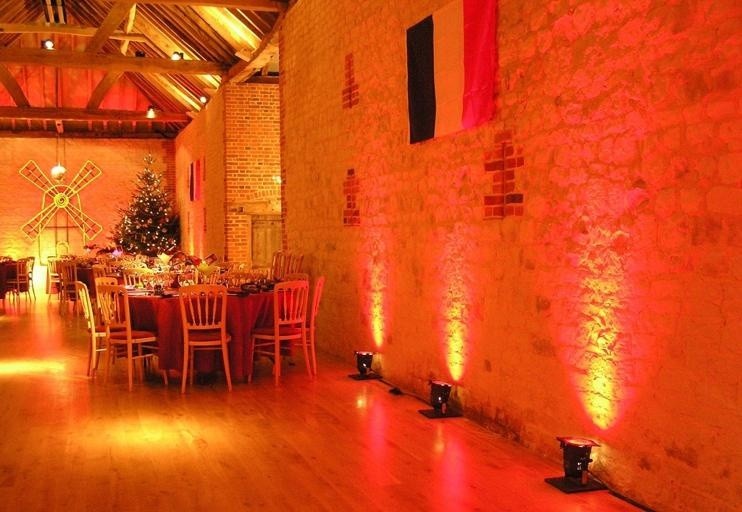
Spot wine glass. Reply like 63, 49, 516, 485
131, 265, 269, 290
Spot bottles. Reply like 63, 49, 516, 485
153, 279, 162, 295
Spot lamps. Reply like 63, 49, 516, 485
145, 104, 156, 119
545, 437, 607, 494
52, 131, 66, 185
171, 52, 183, 60
348, 350, 382, 380
417, 381, 463, 418
40, 39, 54, 49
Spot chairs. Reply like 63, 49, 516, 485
48, 249, 326, 397
1, 256, 36, 314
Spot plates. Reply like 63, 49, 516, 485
226, 280, 277, 297
124, 285, 135, 291
